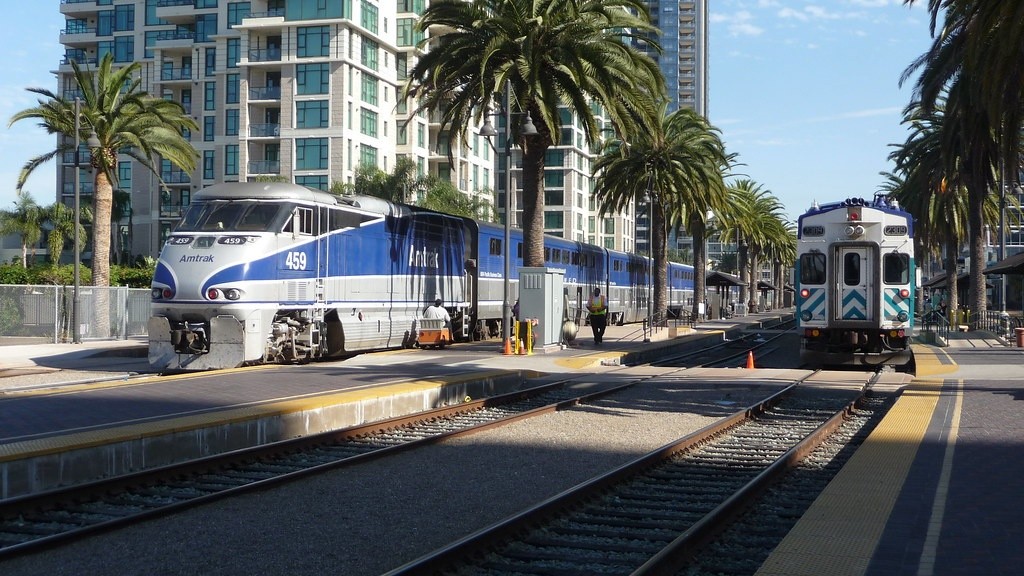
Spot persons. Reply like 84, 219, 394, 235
587, 288, 608, 344
424, 299, 455, 343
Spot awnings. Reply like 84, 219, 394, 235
783, 281, 796, 291
749, 281, 779, 298
706, 272, 748, 306
982, 254, 1024, 274
922, 274, 947, 285
931, 273, 993, 307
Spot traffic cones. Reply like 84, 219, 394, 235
517, 337, 527, 355
502, 338, 515, 355
746, 351, 755, 370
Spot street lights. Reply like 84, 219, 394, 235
639, 178, 662, 329
980, 158, 1024, 335
479, 78, 538, 355
62, 96, 101, 346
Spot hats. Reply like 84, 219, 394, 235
434, 298, 441, 307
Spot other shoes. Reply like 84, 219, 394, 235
593, 332, 603, 345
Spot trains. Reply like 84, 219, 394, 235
146, 181, 761, 372
793, 197, 916, 369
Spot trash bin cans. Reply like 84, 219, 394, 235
1015, 328, 1024, 347
